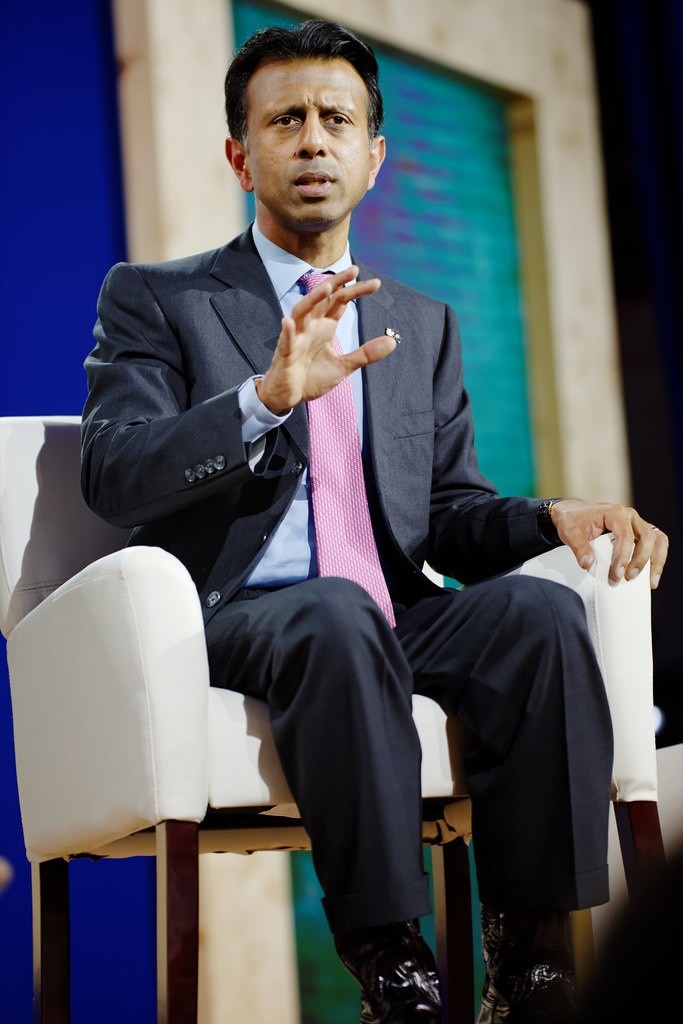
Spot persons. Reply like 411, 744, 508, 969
77, 21, 668, 1024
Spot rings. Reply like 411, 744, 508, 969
652, 527, 659, 530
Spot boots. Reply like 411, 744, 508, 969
477, 907, 580, 1024
334, 916, 444, 1024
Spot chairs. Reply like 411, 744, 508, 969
0, 416, 683, 1024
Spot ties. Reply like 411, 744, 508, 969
298, 273, 396, 630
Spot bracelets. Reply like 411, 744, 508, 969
539, 498, 564, 515
